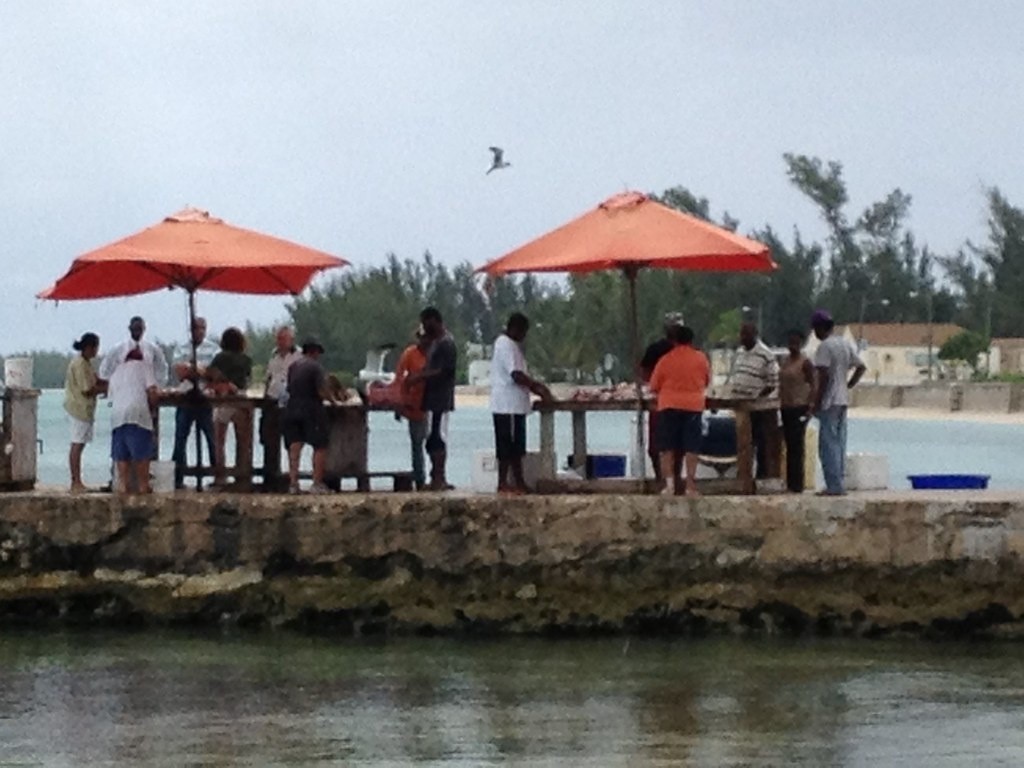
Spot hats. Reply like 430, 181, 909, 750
814, 490, 848, 496
809, 310, 834, 331
300, 334, 327, 354
662, 310, 685, 329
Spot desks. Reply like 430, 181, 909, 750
531, 398, 763, 497
0, 389, 42, 491
158, 393, 367, 491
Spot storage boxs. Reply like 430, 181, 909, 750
478, 448, 557, 495
816, 453, 892, 492
567, 454, 628, 479
908, 472, 992, 489
777, 427, 817, 490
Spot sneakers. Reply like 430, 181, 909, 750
309, 483, 336, 496
288, 485, 307, 495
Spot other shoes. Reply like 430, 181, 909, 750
686, 488, 702, 497
208, 481, 225, 489
174, 483, 188, 489
356, 385, 371, 407
513, 481, 536, 495
496, 483, 527, 497
417, 483, 427, 491
660, 487, 676, 497
69, 487, 95, 495
423, 474, 458, 491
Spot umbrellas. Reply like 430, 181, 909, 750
36, 206, 350, 489
472, 193, 781, 492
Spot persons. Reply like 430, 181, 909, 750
639, 322, 712, 495
779, 325, 819, 493
172, 318, 222, 489
728, 323, 780, 492
204, 328, 254, 488
63, 333, 109, 494
489, 312, 553, 492
811, 311, 866, 495
108, 349, 165, 494
283, 337, 338, 495
394, 306, 457, 492
98, 316, 170, 462
258, 325, 303, 493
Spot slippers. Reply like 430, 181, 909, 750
138, 487, 154, 495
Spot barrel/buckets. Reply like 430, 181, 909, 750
4, 358, 33, 389
907, 473, 991, 489
567, 453, 626, 479
149, 461, 175, 493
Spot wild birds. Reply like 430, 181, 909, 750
487, 146, 512, 176
